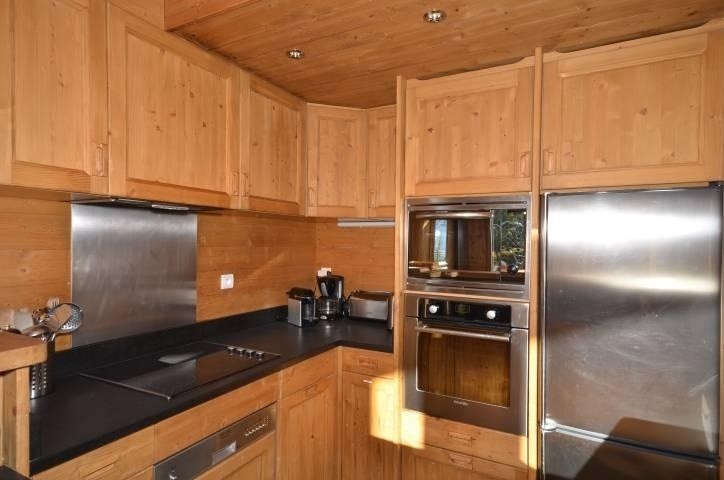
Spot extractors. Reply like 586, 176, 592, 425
72, 198, 228, 214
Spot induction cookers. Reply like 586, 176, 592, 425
80, 340, 281, 400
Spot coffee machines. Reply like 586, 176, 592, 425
285, 287, 317, 327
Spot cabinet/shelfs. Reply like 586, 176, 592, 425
307, 100, 396, 218
108, 5, 299, 206
30, 371, 282, 479
338, 347, 395, 475
0, 0, 108, 200
538, 48, 722, 188
405, 409, 530, 480
282, 344, 337, 479
402, 61, 535, 198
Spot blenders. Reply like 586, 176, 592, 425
317, 275, 346, 322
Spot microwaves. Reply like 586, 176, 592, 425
407, 194, 531, 292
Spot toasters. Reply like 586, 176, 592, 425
347, 290, 395, 323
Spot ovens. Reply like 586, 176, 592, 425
403, 291, 530, 438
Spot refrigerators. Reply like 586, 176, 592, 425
537, 185, 724, 480
